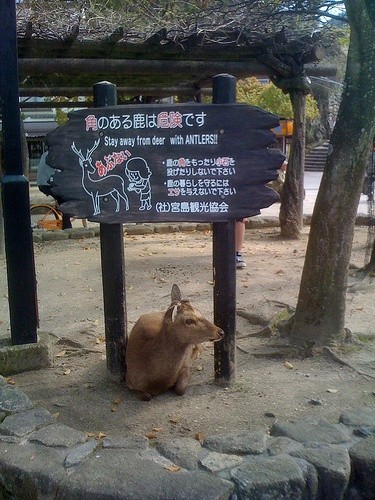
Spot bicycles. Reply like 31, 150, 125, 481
30, 200, 86, 229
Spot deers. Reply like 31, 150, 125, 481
125, 284, 225, 402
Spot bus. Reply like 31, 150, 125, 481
0, 118, 60, 183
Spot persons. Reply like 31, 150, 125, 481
367, 138, 375, 202
268, 141, 288, 172
234, 217, 247, 269
37, 150, 72, 230
